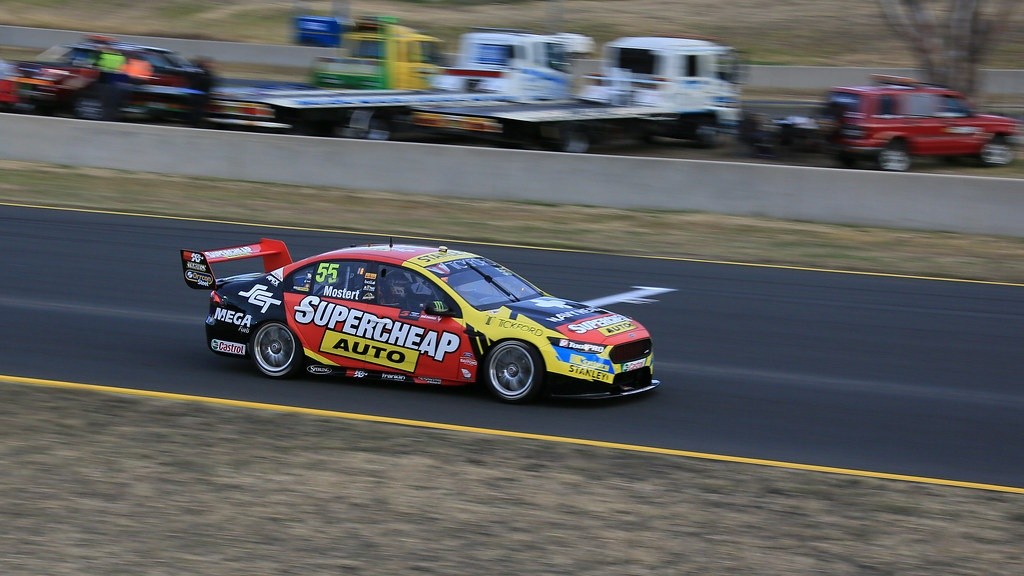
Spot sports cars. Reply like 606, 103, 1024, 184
180, 237, 662, 403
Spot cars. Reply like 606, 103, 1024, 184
0, 43, 214, 121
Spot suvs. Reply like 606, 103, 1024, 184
817, 74, 1022, 174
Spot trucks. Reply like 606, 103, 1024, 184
396, 41, 746, 154
119, 27, 599, 140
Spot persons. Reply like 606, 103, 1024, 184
380, 270, 444, 312
80, 39, 126, 122
